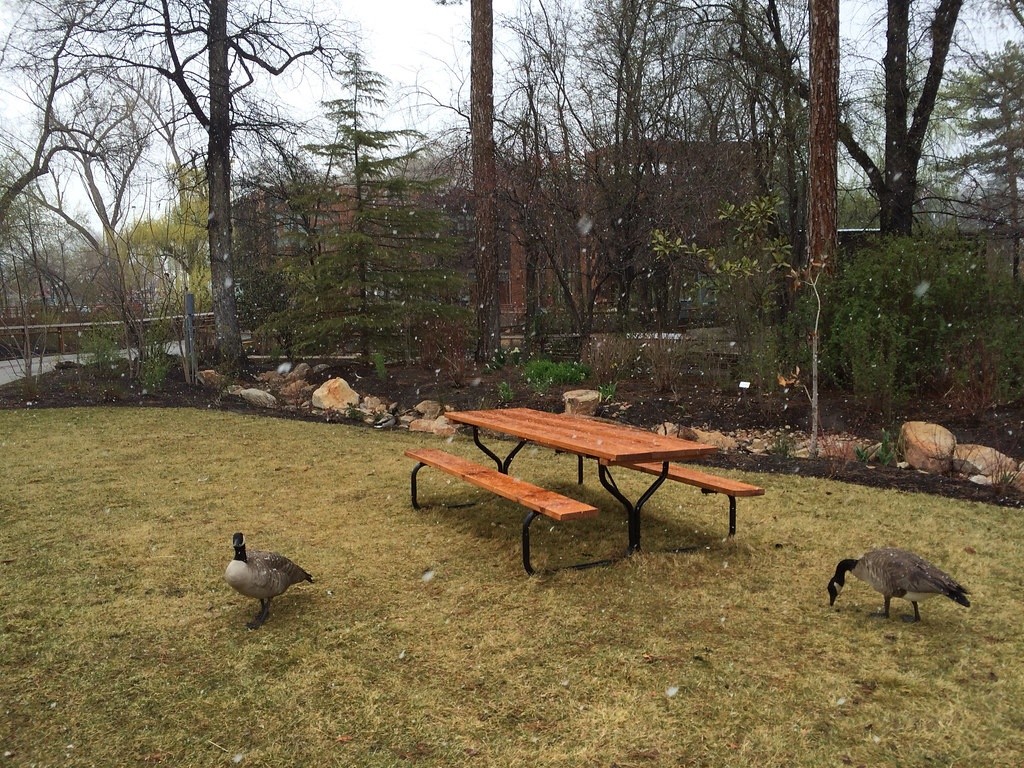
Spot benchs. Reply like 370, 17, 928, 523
404, 450, 597, 574
568, 451, 764, 538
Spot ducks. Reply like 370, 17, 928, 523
827, 549, 971, 623
224, 532, 313, 630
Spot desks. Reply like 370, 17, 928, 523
443, 407, 720, 554
